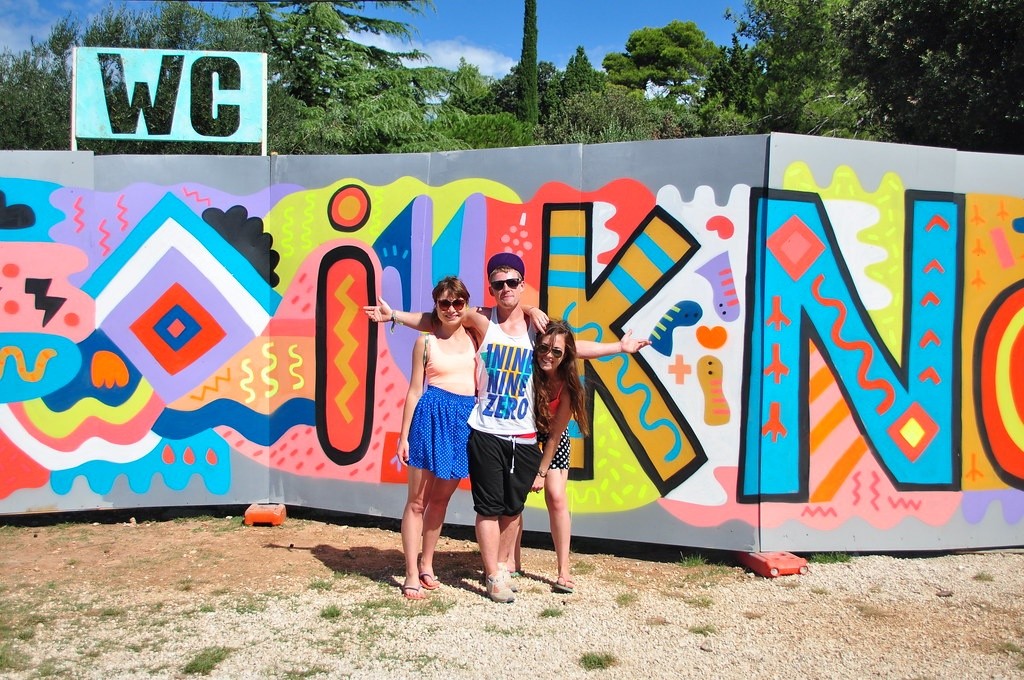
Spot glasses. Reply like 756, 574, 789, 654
437, 299, 467, 311
490, 278, 523, 290
535, 345, 567, 358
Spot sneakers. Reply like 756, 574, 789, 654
498, 563, 517, 591
486, 574, 516, 603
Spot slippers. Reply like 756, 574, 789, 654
510, 559, 520, 575
419, 572, 440, 590
401, 584, 424, 599
555, 576, 573, 592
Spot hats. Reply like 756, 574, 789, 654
487, 253, 524, 278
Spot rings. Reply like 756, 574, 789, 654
536, 491, 539, 493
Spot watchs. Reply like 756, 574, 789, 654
538, 469, 546, 477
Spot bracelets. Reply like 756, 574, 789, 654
390, 309, 402, 334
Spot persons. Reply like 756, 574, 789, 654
507, 318, 591, 594
396, 277, 550, 600
362, 253, 654, 603
